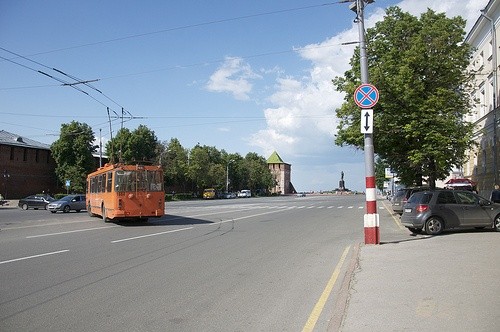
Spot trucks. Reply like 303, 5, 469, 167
444, 178, 478, 195
238, 189, 251, 198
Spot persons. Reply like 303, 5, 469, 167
341, 171, 344, 179
469, 186, 478, 194
491, 185, 500, 203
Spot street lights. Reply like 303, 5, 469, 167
3, 170, 11, 200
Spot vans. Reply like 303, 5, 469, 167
202, 188, 216, 200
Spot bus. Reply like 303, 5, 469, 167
86, 107, 166, 223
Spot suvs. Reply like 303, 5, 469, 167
400, 190, 500, 236
391, 187, 430, 216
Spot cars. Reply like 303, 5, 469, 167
17, 194, 55, 211
47, 194, 86, 214
217, 192, 237, 199
